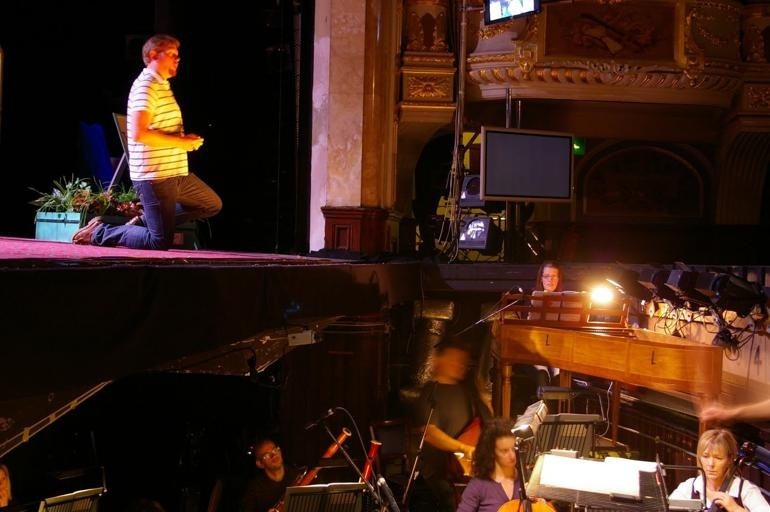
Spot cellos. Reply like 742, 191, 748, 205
498, 436, 558, 511
701, 441, 756, 512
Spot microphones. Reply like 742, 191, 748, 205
304, 408, 335, 431
518, 287, 532, 305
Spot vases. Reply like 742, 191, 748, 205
35, 212, 131, 243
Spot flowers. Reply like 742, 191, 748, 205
26, 172, 146, 228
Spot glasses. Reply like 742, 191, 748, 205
261, 446, 282, 462
542, 275, 558, 280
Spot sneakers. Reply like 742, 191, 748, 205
71, 216, 105, 244
125, 216, 143, 226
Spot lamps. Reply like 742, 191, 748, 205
588, 269, 760, 318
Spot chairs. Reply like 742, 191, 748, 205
369, 415, 422, 511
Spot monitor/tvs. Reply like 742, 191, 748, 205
480, 125, 577, 204
485, 0, 541, 25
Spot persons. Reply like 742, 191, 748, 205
238, 436, 324, 512
0, 463, 14, 512
699, 397, 769, 425
667, 428, 770, 512
70, 34, 224, 252
456, 423, 547, 512
412, 338, 478, 511
536, 260, 565, 294
